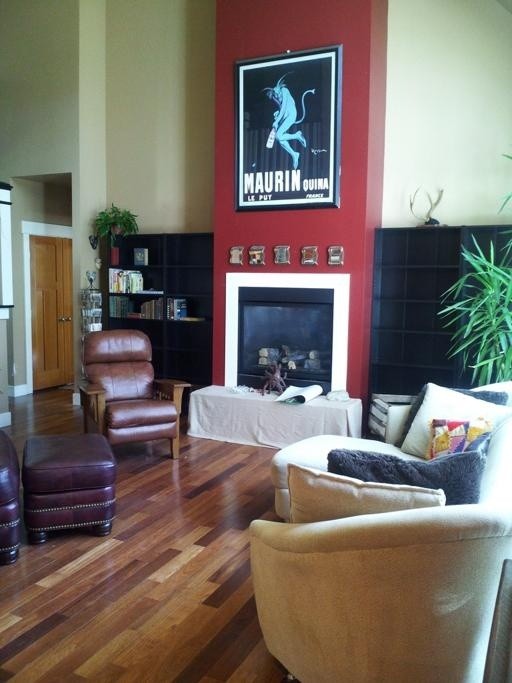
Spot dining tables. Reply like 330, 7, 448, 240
95, 207, 135, 265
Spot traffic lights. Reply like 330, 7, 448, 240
287, 462, 448, 523
329, 449, 484, 507
427, 420, 481, 464
465, 433, 489, 455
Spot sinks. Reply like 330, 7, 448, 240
267, 383, 511, 507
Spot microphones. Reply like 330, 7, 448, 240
0, 430, 23, 562
22, 435, 117, 542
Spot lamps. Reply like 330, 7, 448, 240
76, 331, 190, 459
249, 505, 511, 682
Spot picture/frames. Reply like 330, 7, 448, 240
187, 386, 363, 452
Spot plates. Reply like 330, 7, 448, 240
369, 225, 510, 393
78, 288, 102, 333
108, 234, 212, 385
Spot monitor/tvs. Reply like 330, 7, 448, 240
108, 268, 144, 294
109, 296, 206, 323
134, 247, 149, 266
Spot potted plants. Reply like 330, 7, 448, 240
233, 43, 344, 212
